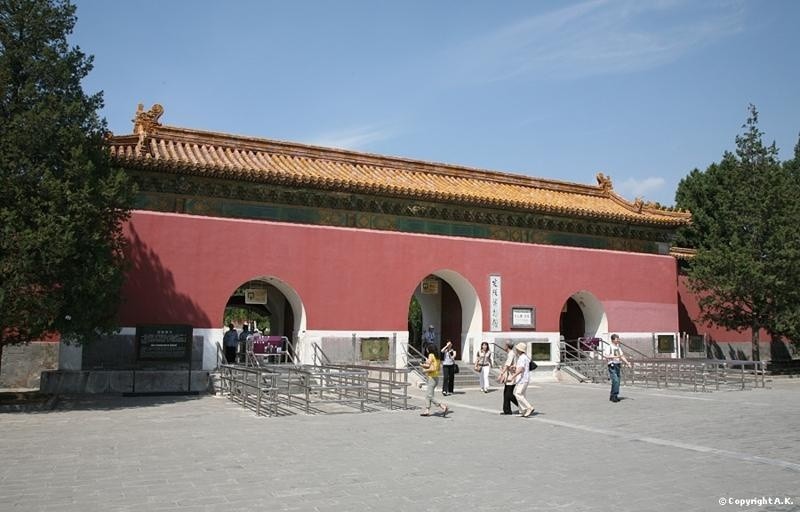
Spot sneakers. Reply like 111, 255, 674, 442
524, 407, 534, 417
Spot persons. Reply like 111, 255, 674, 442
418, 345, 448, 417
239, 325, 252, 360
423, 324, 436, 345
223, 323, 239, 363
475, 341, 493, 394
440, 338, 457, 398
508, 343, 535, 417
496, 339, 519, 415
605, 334, 632, 403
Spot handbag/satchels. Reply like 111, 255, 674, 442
454, 364, 459, 373
474, 364, 482, 372
529, 361, 537, 371
422, 362, 435, 373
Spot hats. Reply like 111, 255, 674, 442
515, 343, 526, 353
